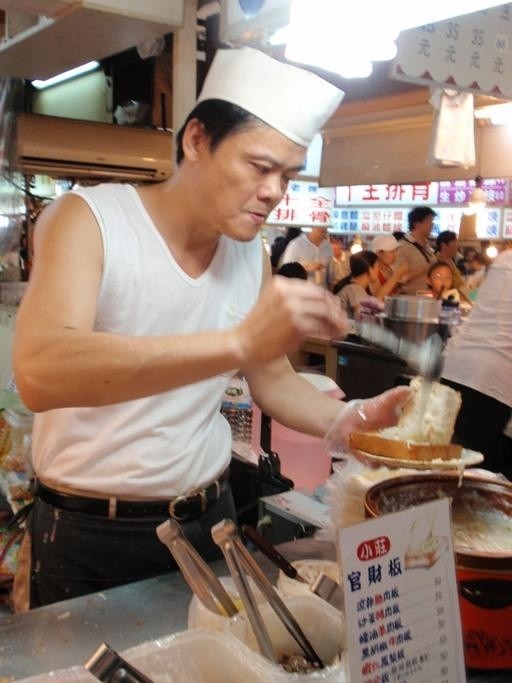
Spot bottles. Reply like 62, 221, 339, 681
442, 297, 462, 325
220, 370, 253, 447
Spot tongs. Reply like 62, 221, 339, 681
158, 521, 236, 616
205, 509, 325, 680
85, 642, 154, 683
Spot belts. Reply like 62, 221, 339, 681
31, 478, 227, 521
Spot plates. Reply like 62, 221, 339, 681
354, 448, 485, 469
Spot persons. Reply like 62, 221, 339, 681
11, 48, 414, 621
429, 243, 511, 486
271, 204, 483, 316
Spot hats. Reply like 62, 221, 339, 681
373, 234, 400, 252
196, 46, 345, 149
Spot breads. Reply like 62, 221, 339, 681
345, 376, 468, 461
404, 508, 449, 570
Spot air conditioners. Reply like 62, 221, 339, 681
9, 113, 174, 183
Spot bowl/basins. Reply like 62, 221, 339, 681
271, 554, 341, 594
186, 576, 273, 629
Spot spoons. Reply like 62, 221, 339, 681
346, 320, 445, 383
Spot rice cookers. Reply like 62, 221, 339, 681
361, 470, 512, 672
